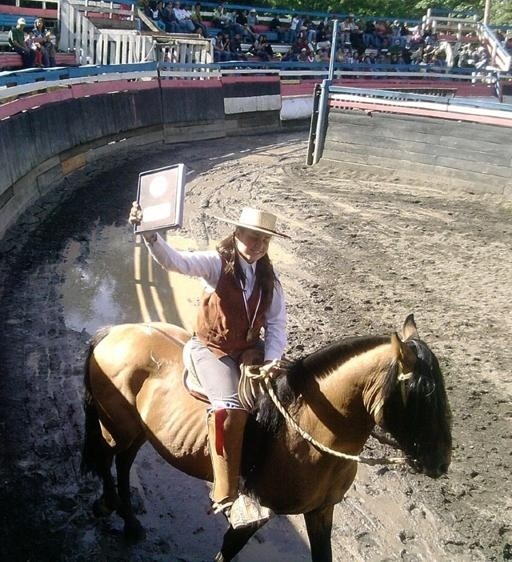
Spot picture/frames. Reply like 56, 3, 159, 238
133, 162, 185, 235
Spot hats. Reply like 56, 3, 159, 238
17, 17, 26, 25
215, 207, 291, 239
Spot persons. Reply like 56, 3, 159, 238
7, 17, 38, 69
143, 0, 489, 81
25, 28, 46, 68
128, 198, 289, 534
31, 16, 58, 68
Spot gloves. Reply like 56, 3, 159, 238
129, 200, 156, 243
264, 359, 279, 377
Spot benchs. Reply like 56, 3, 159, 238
1, 4, 480, 69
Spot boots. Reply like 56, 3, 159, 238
205, 406, 273, 525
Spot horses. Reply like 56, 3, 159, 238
78, 313, 453, 562
